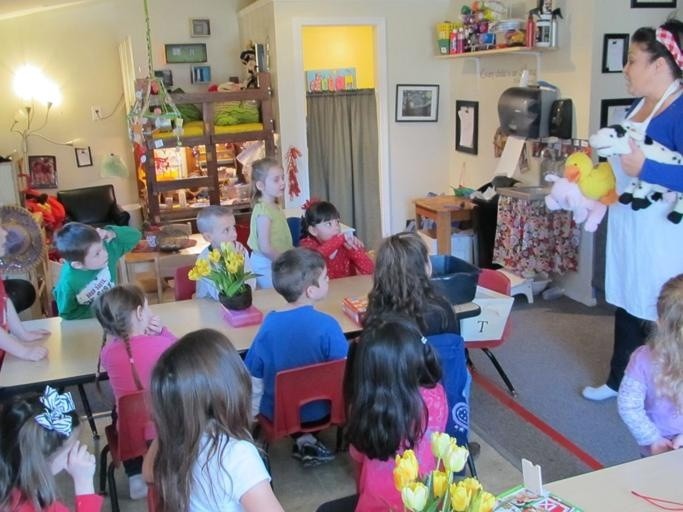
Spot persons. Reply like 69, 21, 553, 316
0, 385, 104, 512
51, 221, 143, 321
140, 329, 285, 512
92, 286, 179, 502
243, 246, 350, 470
361, 231, 481, 456
195, 205, 257, 301
0, 276, 52, 369
246, 158, 296, 289
581, 18, 683, 402
616, 272, 682, 459
345, 311, 450, 512
298, 201, 375, 281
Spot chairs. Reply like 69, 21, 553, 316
43, 221, 210, 315
100, 389, 160, 511
286, 217, 307, 248
53, 182, 132, 230
147, 484, 167, 512
255, 358, 346, 459
461, 267, 518, 399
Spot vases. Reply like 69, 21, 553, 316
220, 284, 251, 311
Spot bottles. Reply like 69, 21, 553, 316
449, 29, 457, 55
534, 19, 552, 46
178, 188, 187, 209
460, 0, 507, 45
456, 27, 466, 54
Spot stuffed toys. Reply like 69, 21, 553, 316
544, 124, 682, 233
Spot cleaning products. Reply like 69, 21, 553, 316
549, 7, 564, 47
526, 6, 542, 48
448, 27, 463, 56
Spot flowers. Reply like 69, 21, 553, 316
393, 432, 497, 512
189, 244, 262, 296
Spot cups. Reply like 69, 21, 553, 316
165, 197, 174, 211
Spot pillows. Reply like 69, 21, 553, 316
211, 104, 261, 129
160, 87, 202, 125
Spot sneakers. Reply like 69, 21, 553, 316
126, 470, 149, 501
582, 381, 618, 404
290, 439, 336, 469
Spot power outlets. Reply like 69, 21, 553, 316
90, 106, 104, 120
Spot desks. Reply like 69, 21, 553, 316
504, 446, 683, 509
338, 221, 358, 239
413, 197, 478, 262
0, 272, 481, 439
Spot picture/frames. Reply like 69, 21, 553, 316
74, 147, 93, 167
629, 0, 677, 11
393, 84, 441, 125
601, 33, 630, 74
26, 155, 57, 192
597, 97, 641, 163
453, 99, 479, 155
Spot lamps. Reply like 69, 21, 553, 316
7, 63, 82, 187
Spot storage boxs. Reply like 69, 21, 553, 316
426, 253, 481, 304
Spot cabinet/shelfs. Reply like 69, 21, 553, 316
122, 204, 145, 232
